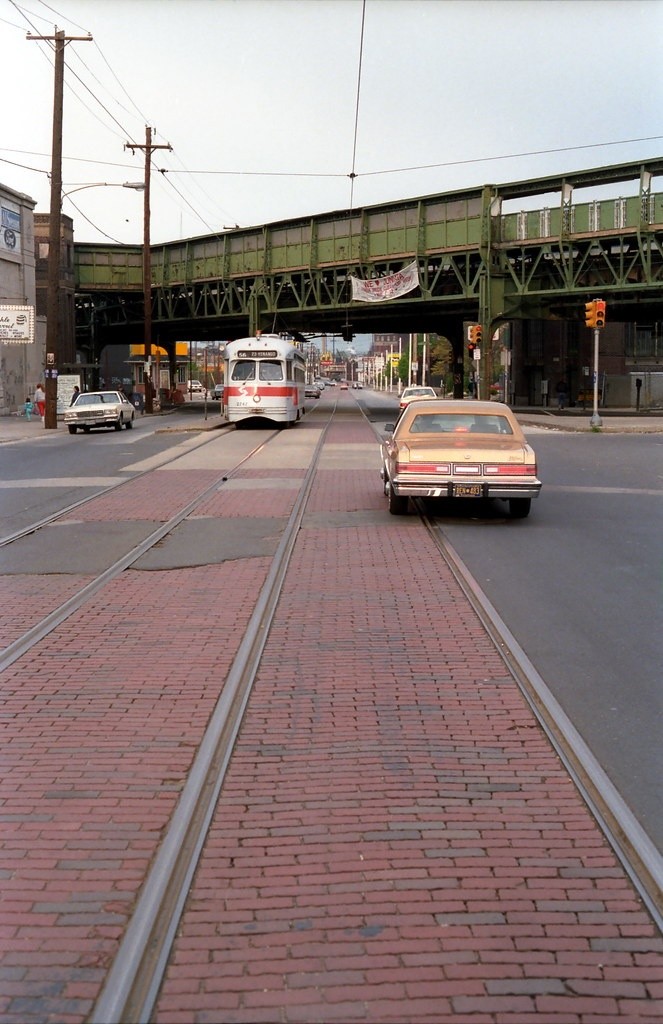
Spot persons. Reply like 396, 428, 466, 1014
24, 398, 34, 422
70, 386, 80, 407
555, 376, 567, 409
35, 382, 47, 423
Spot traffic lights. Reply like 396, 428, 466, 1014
468, 326, 474, 341
473, 326, 482, 344
596, 301, 604, 328
584, 300, 596, 328
468, 343, 476, 358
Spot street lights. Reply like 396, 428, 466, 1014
45, 180, 148, 429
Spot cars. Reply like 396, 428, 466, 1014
305, 385, 320, 399
312, 380, 364, 391
379, 399, 542, 519
210, 384, 224, 400
63, 391, 136, 434
188, 380, 203, 393
397, 386, 438, 415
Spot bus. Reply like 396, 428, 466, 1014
222, 330, 306, 431
